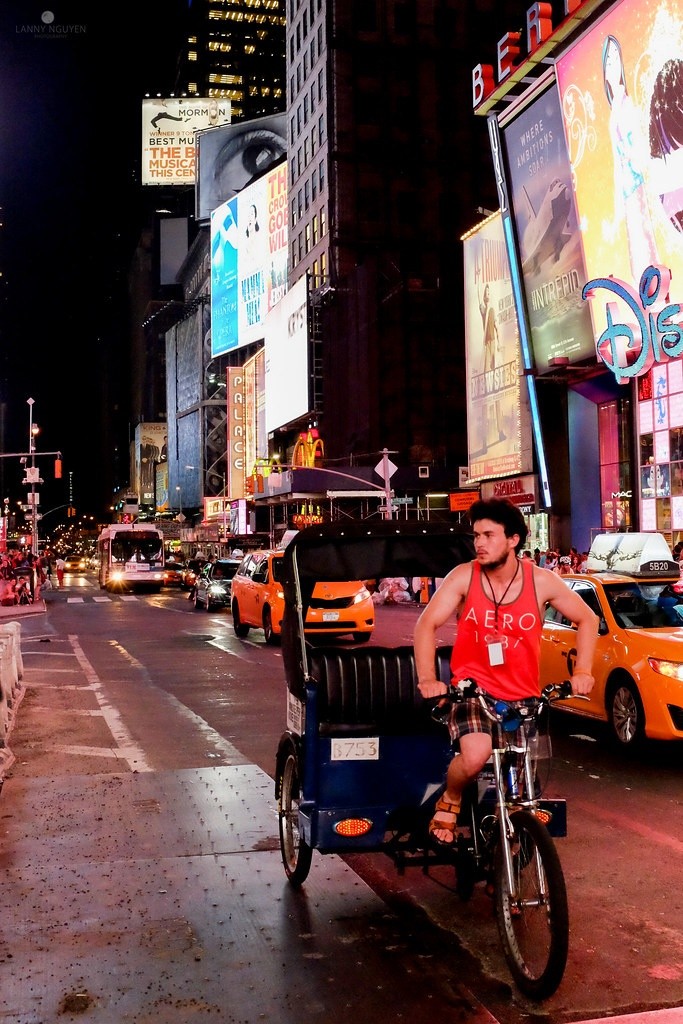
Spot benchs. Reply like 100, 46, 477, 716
296, 646, 453, 745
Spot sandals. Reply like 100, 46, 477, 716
485, 883, 523, 919
429, 795, 461, 849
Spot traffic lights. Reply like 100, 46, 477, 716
245, 475, 255, 495
55, 460, 62, 478
71, 507, 76, 516
257, 475, 264, 494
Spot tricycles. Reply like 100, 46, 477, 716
272, 520, 591, 1005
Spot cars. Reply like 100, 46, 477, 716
180, 552, 211, 591
534, 532, 682, 755
89, 554, 98, 570
64, 555, 86, 573
163, 556, 185, 587
193, 560, 242, 612
231, 530, 375, 646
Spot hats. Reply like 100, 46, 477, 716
551, 552, 558, 557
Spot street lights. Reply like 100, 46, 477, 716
184, 465, 226, 559
31, 422, 39, 556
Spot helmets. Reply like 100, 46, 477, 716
168, 556, 175, 563
231, 549, 244, 560
195, 551, 205, 561
639, 585, 661, 601
560, 556, 571, 563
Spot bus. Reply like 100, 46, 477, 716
98, 524, 164, 592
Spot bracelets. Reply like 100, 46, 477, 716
572, 667, 591, 677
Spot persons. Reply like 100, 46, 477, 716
476, 275, 508, 449
0, 547, 68, 609
411, 500, 599, 847
522, 541, 683, 575
